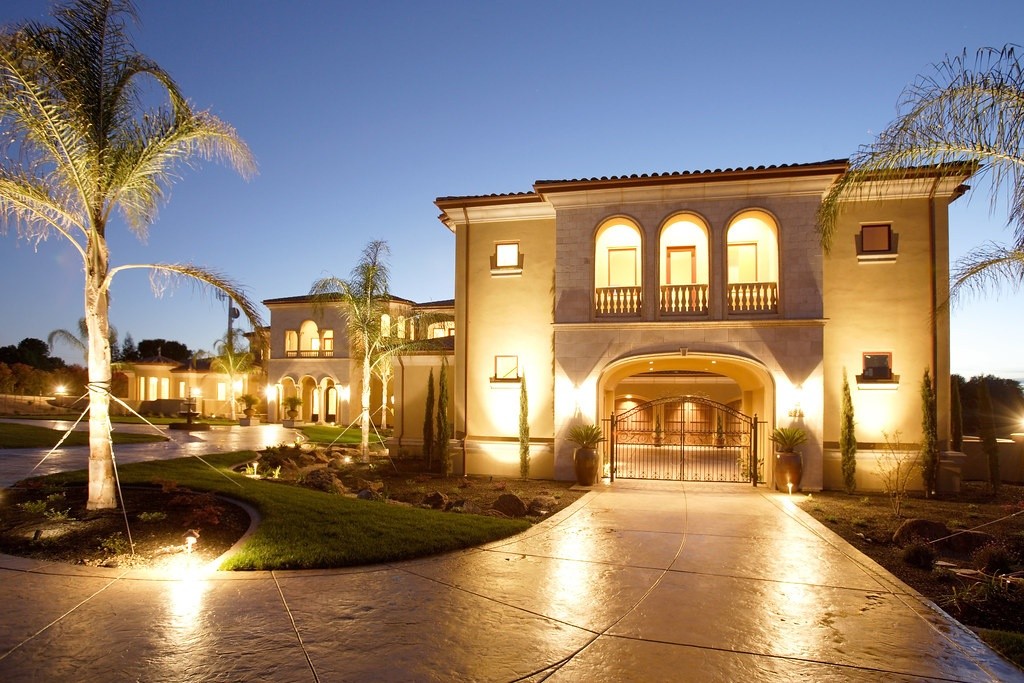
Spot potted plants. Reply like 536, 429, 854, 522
567, 425, 608, 486
768, 427, 807, 492
653, 414, 663, 448
716, 415, 726, 449
281, 396, 303, 420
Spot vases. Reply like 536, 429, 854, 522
235, 393, 261, 418
